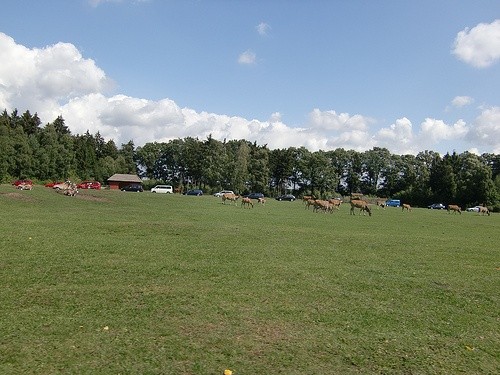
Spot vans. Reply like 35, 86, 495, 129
385, 199, 400, 208
151, 184, 173, 195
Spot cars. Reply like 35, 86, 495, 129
466, 206, 482, 212
13, 178, 33, 191
44, 181, 78, 191
76, 180, 101, 190
275, 194, 296, 201
427, 203, 446, 210
121, 184, 143, 193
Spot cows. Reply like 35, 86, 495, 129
241, 198, 253, 209
350, 200, 371, 216
258, 198, 266, 205
376, 201, 386, 210
19, 180, 81, 197
447, 205, 462, 215
478, 207, 490, 216
402, 204, 412, 214
303, 194, 344, 214
222, 194, 240, 205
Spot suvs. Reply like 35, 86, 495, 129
243, 192, 265, 199
182, 189, 203, 196
213, 190, 235, 198
